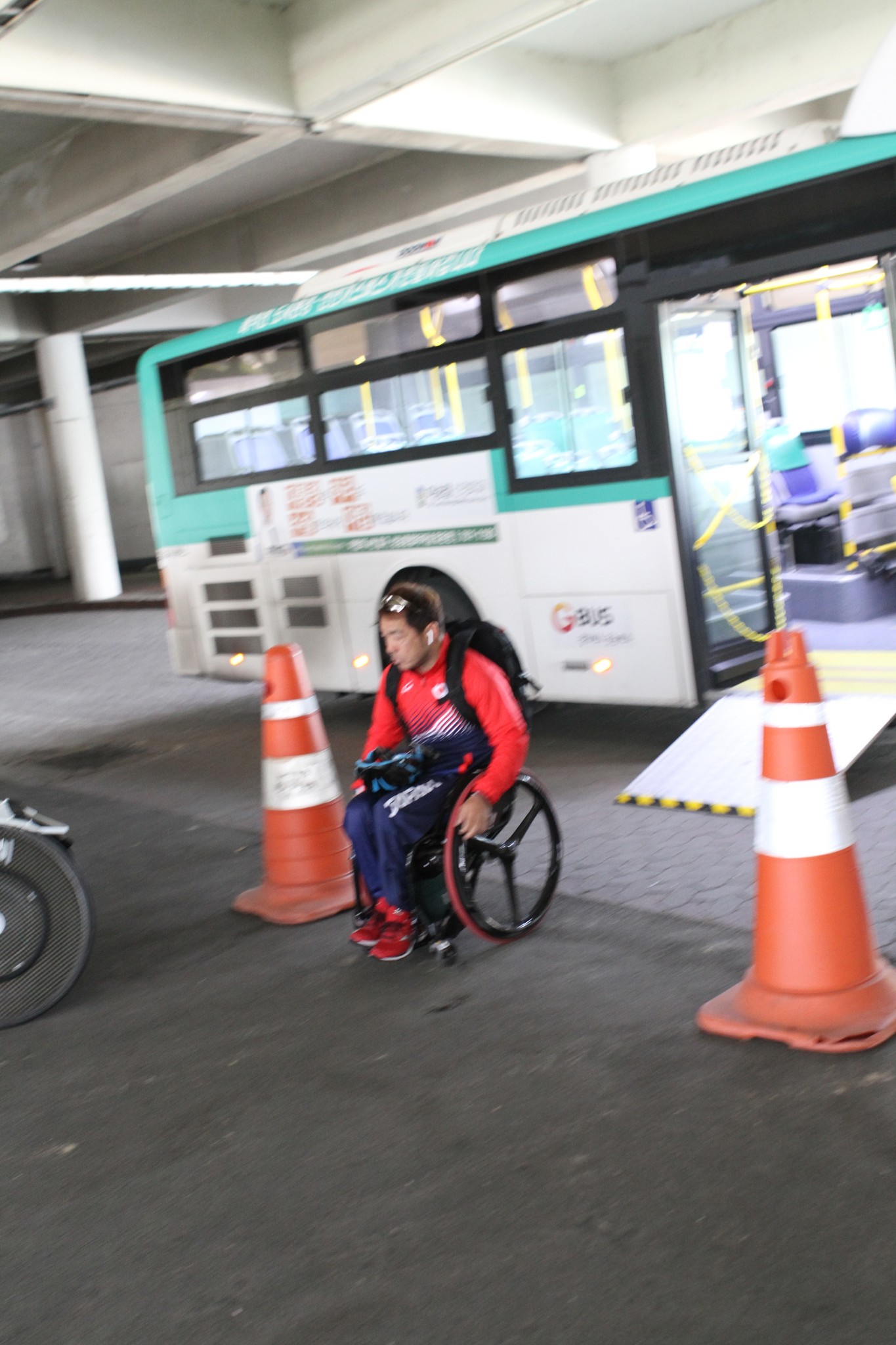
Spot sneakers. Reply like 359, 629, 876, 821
366, 905, 429, 962
349, 898, 390, 949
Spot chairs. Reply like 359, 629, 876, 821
198, 405, 896, 524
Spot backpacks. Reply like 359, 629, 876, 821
386, 621, 532, 741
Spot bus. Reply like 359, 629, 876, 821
137, 29, 895, 712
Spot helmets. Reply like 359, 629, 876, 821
353, 747, 427, 800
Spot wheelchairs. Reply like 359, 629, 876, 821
349, 670, 563, 964
1, 793, 92, 1028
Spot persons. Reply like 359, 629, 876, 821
341, 575, 528, 960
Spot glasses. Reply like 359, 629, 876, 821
378, 594, 427, 619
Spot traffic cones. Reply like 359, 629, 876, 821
694, 629, 896, 1054
231, 643, 367, 927
